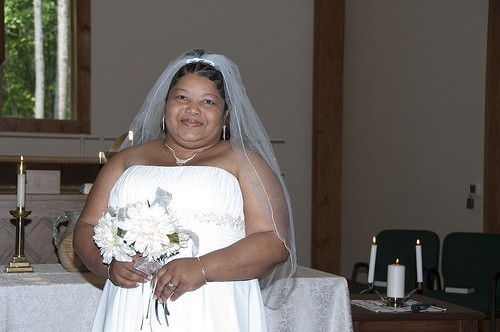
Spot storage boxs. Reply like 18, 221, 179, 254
25, 170, 62, 195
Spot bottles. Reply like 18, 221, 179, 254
52, 210, 93, 272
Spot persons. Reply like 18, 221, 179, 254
73, 48, 297, 332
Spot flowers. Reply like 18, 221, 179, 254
92, 200, 190, 265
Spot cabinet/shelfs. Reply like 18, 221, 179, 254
0, 154, 107, 264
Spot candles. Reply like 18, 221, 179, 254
17, 154, 27, 208
368, 236, 378, 283
387, 259, 405, 299
416, 239, 424, 283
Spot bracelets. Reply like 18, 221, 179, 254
107, 263, 111, 279
197, 256, 207, 285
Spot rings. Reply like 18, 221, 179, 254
167, 281, 177, 288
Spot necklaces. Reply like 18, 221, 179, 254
165, 141, 197, 166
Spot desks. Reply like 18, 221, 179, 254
0, 263, 354, 332
349, 292, 491, 332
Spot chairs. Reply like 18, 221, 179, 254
417, 230, 500, 332
352, 229, 440, 295
